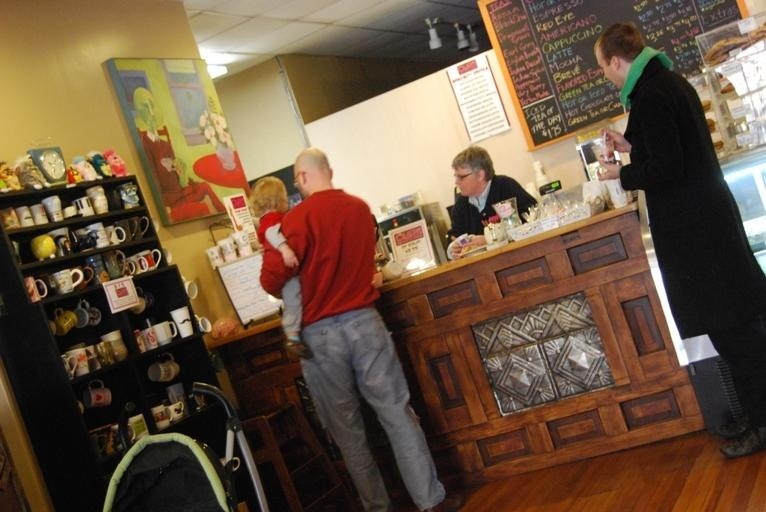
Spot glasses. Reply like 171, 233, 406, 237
455, 172, 473, 179
292, 171, 309, 190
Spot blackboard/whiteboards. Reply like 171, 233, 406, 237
477, 0, 751, 152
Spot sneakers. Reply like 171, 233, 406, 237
284, 339, 313, 359
715, 414, 752, 438
720, 426, 766, 456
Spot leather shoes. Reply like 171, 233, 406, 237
426, 494, 465, 512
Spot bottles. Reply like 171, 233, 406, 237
524, 160, 555, 204
399, 194, 417, 209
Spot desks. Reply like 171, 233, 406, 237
210, 198, 706, 492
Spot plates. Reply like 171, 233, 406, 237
462, 245, 486, 258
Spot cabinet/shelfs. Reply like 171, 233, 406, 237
0, 173, 223, 512
695, 12, 766, 162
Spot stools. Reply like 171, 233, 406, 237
240, 402, 356, 511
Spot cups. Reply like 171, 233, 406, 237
205, 230, 253, 271
589, 135, 633, 211
0, 181, 212, 448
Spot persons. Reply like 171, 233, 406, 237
445, 145, 539, 260
259, 147, 468, 512
593, 21, 765, 459
247, 176, 314, 362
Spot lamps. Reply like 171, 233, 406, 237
425, 18, 443, 52
454, 23, 469, 51
466, 26, 480, 53
